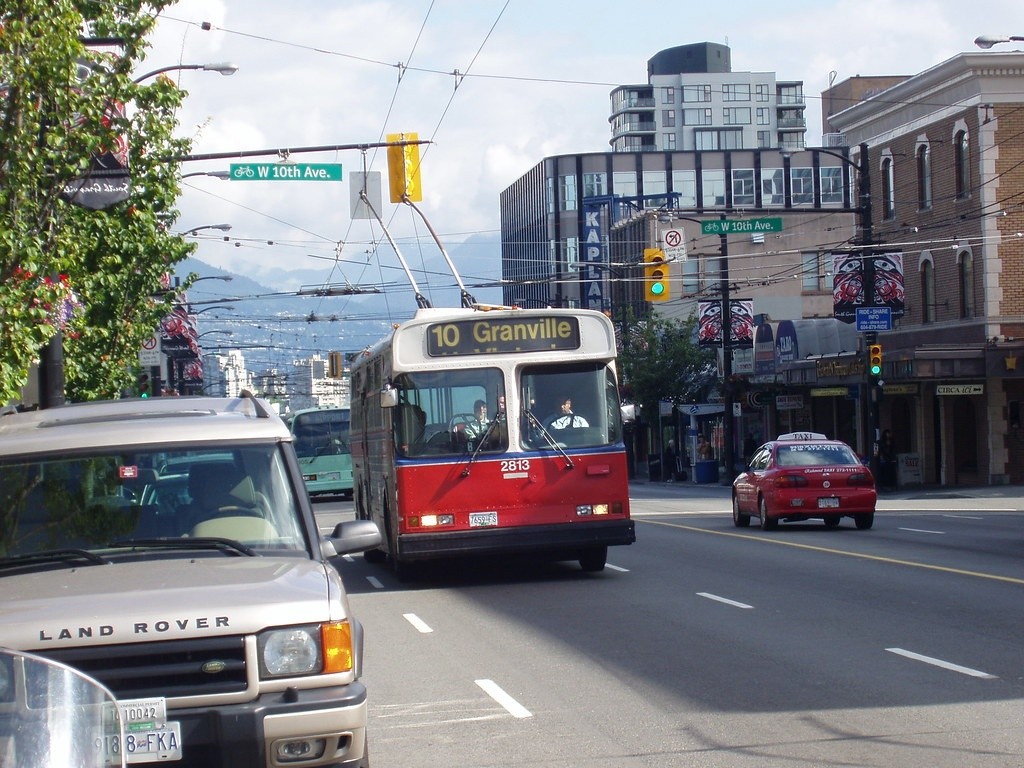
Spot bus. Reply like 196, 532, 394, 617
348, 190, 638, 584
286, 403, 354, 496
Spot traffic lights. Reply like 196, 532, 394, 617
643, 248, 671, 302
137, 374, 150, 398
869, 344, 883, 376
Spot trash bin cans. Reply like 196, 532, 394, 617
696, 461, 718, 484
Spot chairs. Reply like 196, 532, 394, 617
167, 463, 268, 539
10, 478, 100, 555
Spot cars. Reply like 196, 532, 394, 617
731, 431, 877, 531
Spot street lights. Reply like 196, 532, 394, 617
569, 261, 636, 481
778, 142, 880, 493
39, 59, 242, 524
655, 214, 736, 486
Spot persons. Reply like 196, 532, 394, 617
696, 431, 711, 459
394, 401, 451, 454
464, 399, 499, 441
538, 394, 589, 437
744, 432, 757, 471
665, 439, 674, 456
878, 429, 898, 489
841, 437, 871, 467
498, 392, 507, 420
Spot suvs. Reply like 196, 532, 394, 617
0, 385, 384, 768
